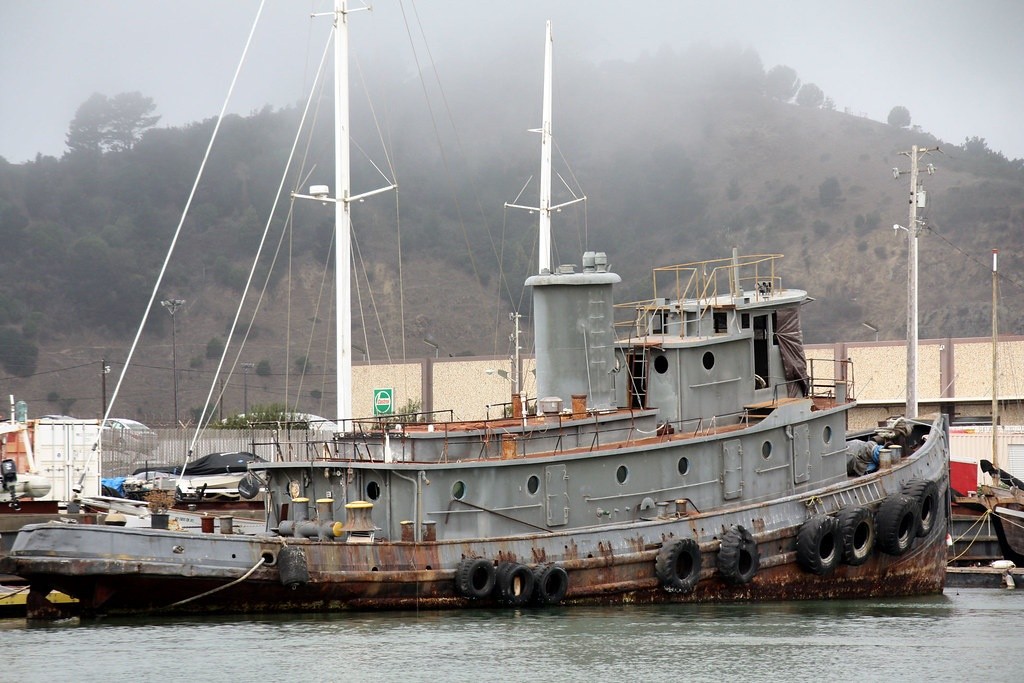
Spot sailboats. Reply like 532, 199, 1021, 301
7, 0, 951, 613
947, 247, 1024, 587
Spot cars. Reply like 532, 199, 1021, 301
99, 419, 159, 452
34, 414, 76, 420
220, 412, 337, 432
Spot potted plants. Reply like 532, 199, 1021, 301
143, 490, 175, 529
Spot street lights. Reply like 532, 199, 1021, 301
161, 299, 185, 427
